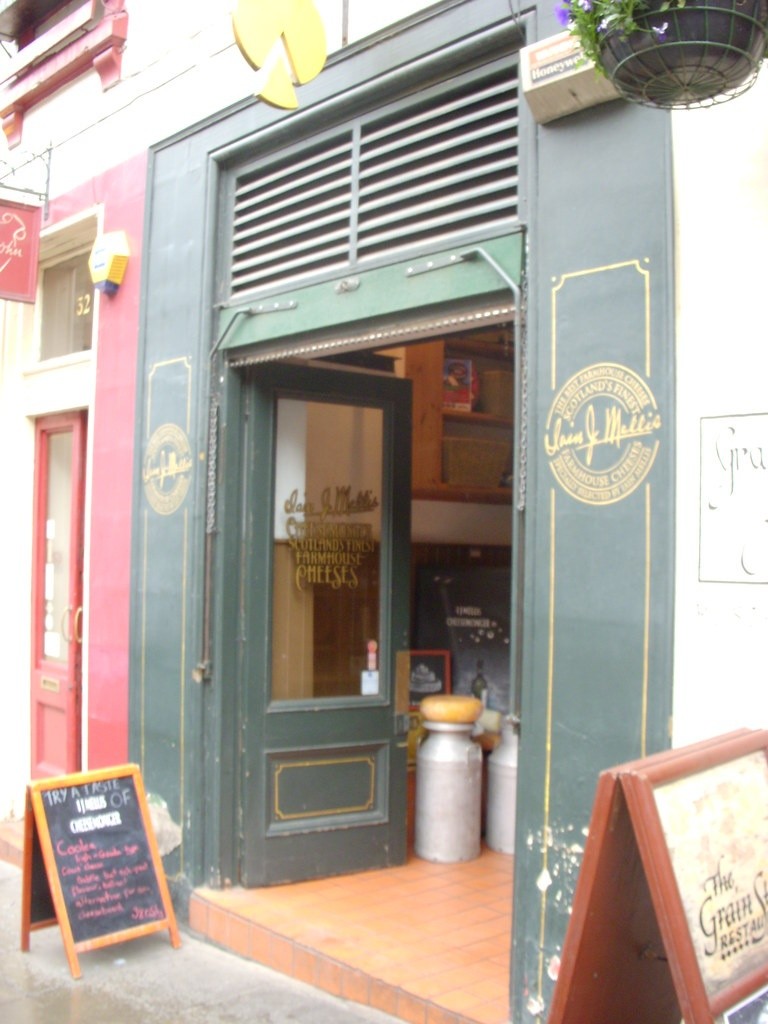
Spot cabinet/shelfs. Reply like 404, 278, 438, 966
411, 336, 514, 504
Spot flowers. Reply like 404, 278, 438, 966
555, 0, 686, 84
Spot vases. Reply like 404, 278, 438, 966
602, 0, 768, 106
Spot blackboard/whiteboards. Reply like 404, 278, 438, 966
19, 763, 175, 952
